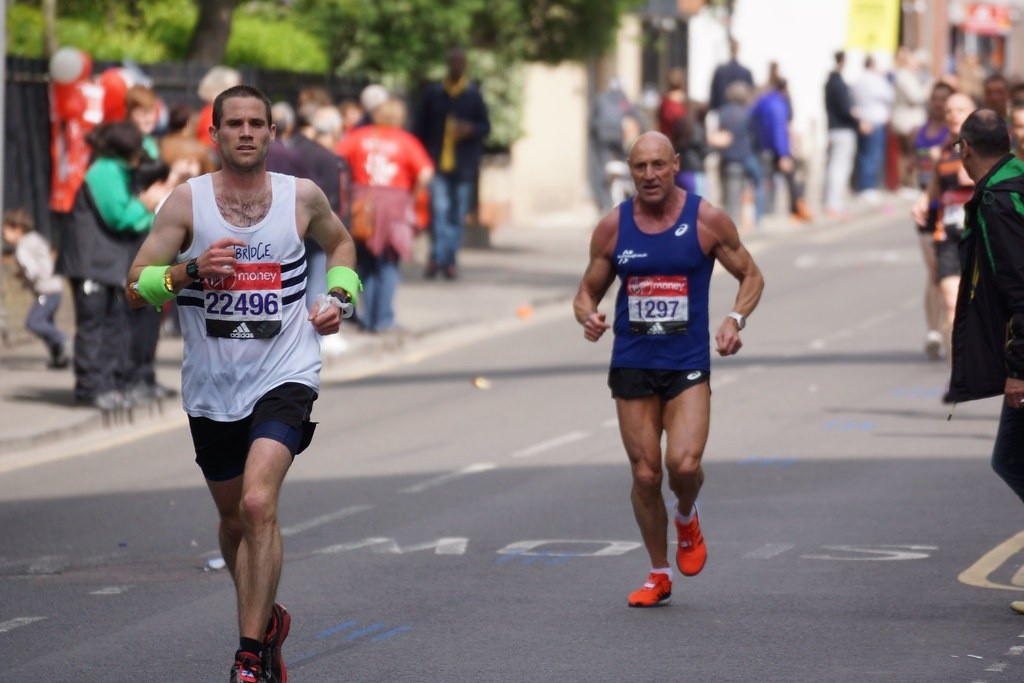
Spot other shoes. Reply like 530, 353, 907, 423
48, 337, 71, 371
422, 258, 461, 282
137, 383, 178, 399
91, 385, 150, 410
925, 331, 943, 362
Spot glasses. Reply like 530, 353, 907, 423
952, 139, 964, 154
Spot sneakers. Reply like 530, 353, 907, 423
230, 603, 291, 683
628, 572, 673, 606
673, 502, 705, 575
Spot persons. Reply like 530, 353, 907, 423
0, 76, 491, 413
124, 87, 362, 683
574, 129, 765, 608
904, 74, 1024, 616
586, 34, 1024, 233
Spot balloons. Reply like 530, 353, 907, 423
48, 45, 90, 89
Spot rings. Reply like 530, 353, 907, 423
1019, 399, 1024, 403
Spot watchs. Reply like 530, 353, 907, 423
727, 311, 747, 332
185, 258, 202, 286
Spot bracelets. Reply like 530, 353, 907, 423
327, 292, 353, 312
